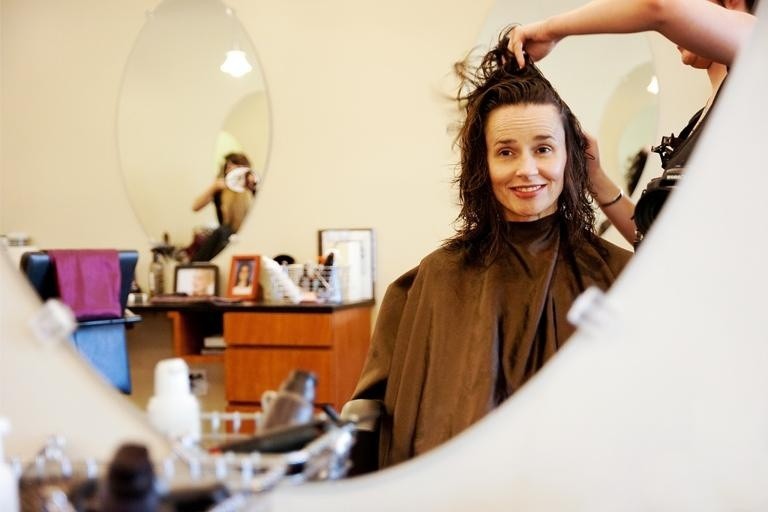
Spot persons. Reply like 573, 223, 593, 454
232, 264, 252, 296
192, 154, 256, 234
501, 0, 758, 247
351, 54, 634, 470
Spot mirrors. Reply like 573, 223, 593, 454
0, 2, 760, 481
115, 3, 274, 264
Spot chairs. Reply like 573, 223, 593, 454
19, 248, 142, 394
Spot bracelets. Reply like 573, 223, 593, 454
601, 189, 623, 208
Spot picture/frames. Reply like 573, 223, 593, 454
226, 255, 259, 305
317, 227, 376, 303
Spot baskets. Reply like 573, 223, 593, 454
162, 410, 347, 495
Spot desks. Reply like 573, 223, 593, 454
127, 289, 377, 434
